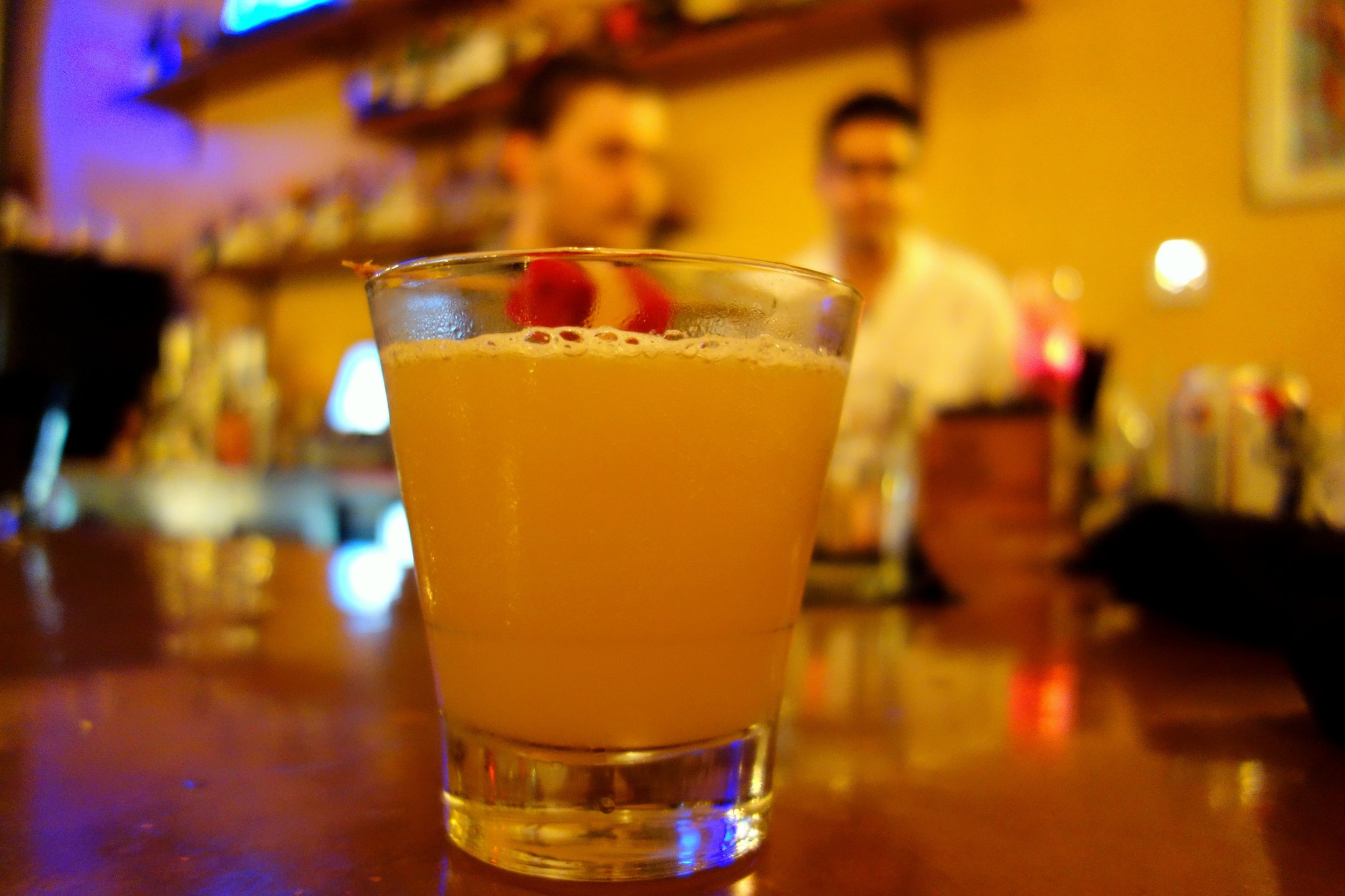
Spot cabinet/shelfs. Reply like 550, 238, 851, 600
137, 0, 1027, 283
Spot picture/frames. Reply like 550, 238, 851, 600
1241, 0, 1345, 211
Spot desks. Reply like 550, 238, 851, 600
0, 544, 1344, 895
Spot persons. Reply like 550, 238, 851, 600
487, 53, 672, 264
732, 92, 1021, 555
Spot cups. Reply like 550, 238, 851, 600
359, 247, 862, 881
